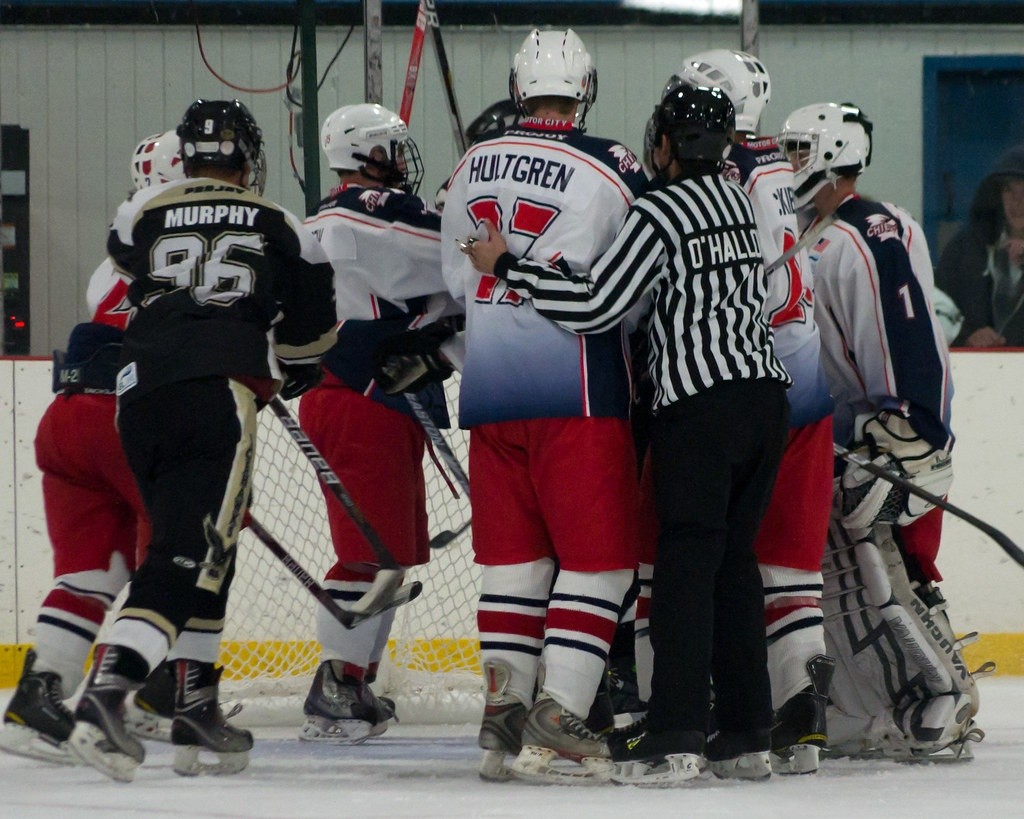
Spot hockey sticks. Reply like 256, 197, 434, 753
270, 396, 401, 616
398, 0, 471, 161
407, 391, 471, 498
251, 518, 424, 631
831, 439, 1024, 564
766, 208, 842, 274
429, 519, 475, 549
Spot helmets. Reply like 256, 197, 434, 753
777, 102, 873, 211
320, 102, 409, 171
177, 98, 262, 174
678, 49, 772, 132
131, 129, 187, 191
513, 27, 593, 103
650, 85, 737, 171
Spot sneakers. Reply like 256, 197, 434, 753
704, 704, 774, 778
604, 714, 705, 787
0, 647, 89, 764
299, 659, 399, 746
475, 658, 535, 781
168, 658, 253, 777
67, 641, 151, 783
509, 657, 612, 786
121, 652, 176, 743
769, 654, 836, 774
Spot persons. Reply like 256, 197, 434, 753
442, 28, 651, 785
777, 101, 996, 763
662, 49, 834, 776
291, 104, 465, 746
938, 147, 1024, 347
470, 85, 792, 785
2, 132, 189, 765
72, 100, 337, 781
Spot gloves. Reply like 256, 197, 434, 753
864, 410, 952, 527
274, 358, 327, 402
383, 337, 455, 396
